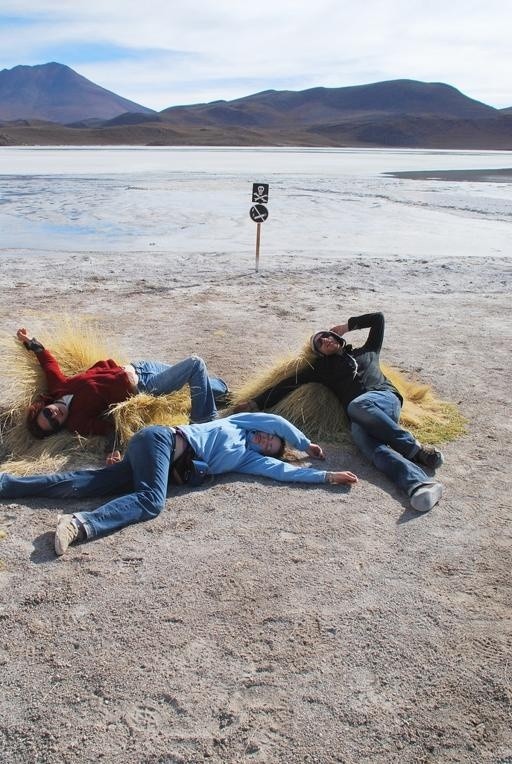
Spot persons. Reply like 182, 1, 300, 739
234, 313, 444, 512
14, 328, 232, 465
2, 409, 358, 556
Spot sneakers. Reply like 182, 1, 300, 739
410, 482, 443, 511
55, 513, 78, 556
412, 443, 445, 468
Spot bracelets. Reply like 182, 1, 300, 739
329, 473, 334, 483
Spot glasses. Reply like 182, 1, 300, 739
43, 407, 60, 431
316, 333, 329, 347
